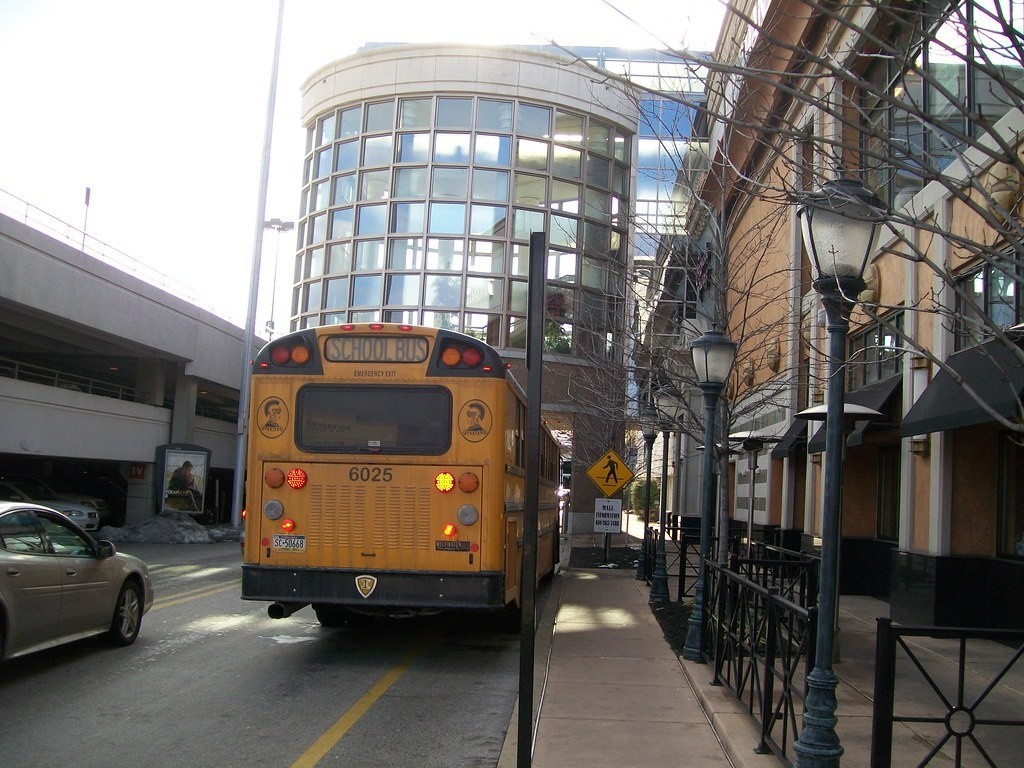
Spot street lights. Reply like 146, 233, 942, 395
727, 430, 785, 584
264, 217, 293, 344
681, 321, 738, 661
791, 154, 890, 768
635, 380, 680, 609
794, 402, 884, 665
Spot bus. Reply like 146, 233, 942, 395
241, 324, 562, 625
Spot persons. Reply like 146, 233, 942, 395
168, 461, 203, 511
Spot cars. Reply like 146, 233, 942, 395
0, 502, 154, 666
0, 475, 112, 531
